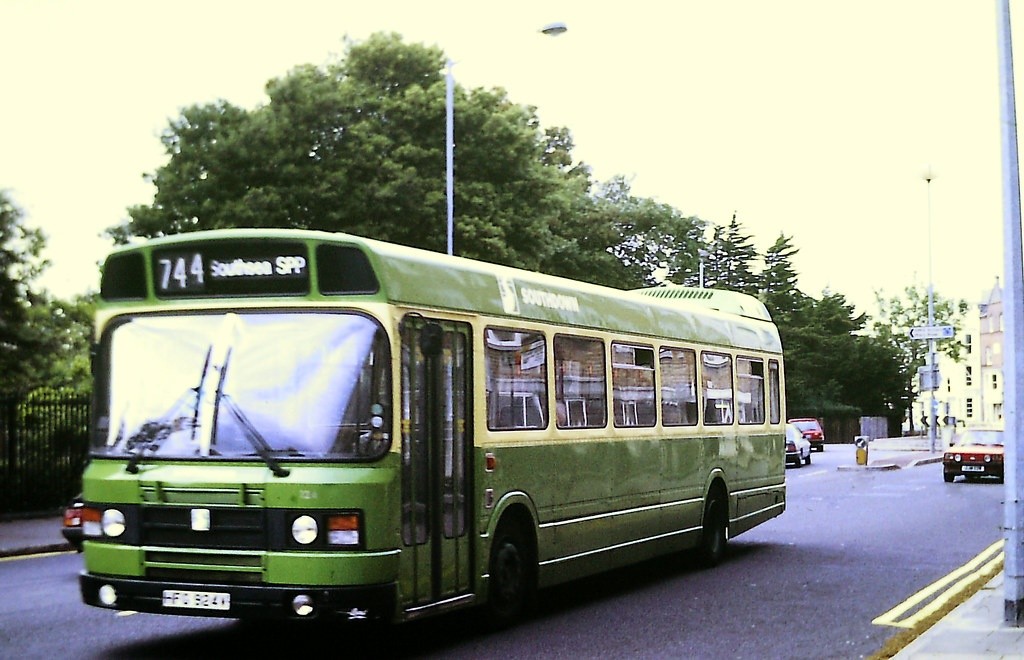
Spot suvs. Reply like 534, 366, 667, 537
786, 418, 825, 453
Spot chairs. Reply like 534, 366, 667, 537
489, 395, 753, 430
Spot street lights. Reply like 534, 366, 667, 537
445, 22, 568, 255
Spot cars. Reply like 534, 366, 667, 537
943, 424, 1004, 484
786, 423, 811, 468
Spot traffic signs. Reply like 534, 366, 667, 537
908, 325, 954, 340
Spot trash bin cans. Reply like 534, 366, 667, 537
940, 427, 952, 453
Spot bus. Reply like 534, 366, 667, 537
75, 228, 788, 626
